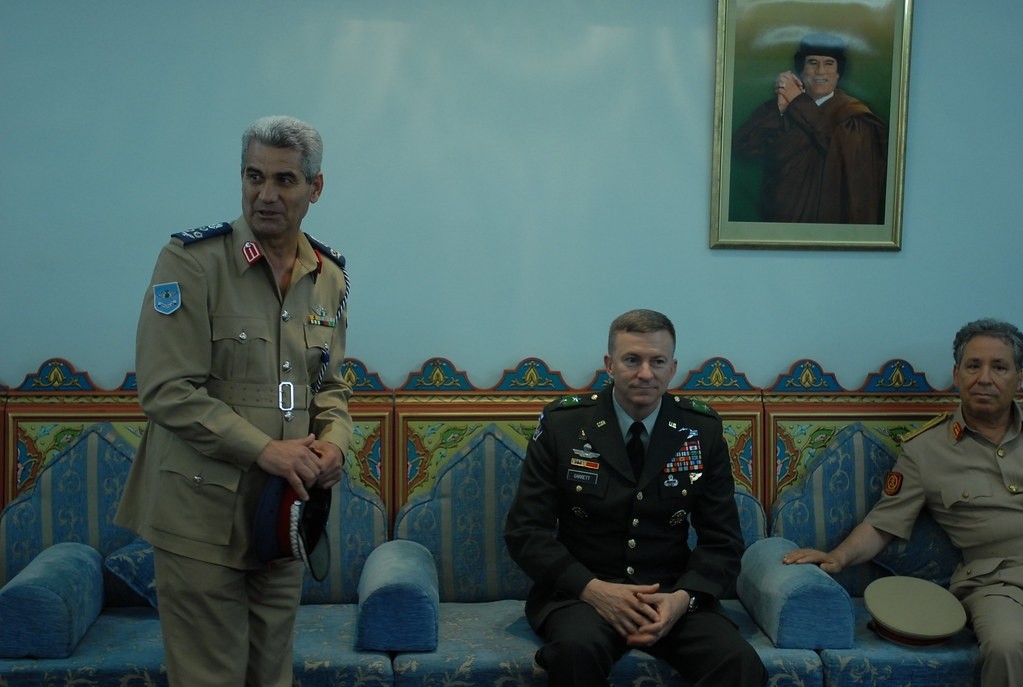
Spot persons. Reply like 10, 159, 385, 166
783, 317, 1023, 687
504, 309, 768, 686
113, 116, 351, 687
730, 34, 886, 224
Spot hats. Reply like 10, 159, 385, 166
249, 448, 332, 580
864, 575, 967, 647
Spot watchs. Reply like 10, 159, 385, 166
683, 589, 699, 615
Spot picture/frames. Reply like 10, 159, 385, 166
708, 0, 916, 253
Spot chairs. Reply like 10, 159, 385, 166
0, 351, 1023, 687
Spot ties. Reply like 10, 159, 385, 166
626, 422, 646, 479
